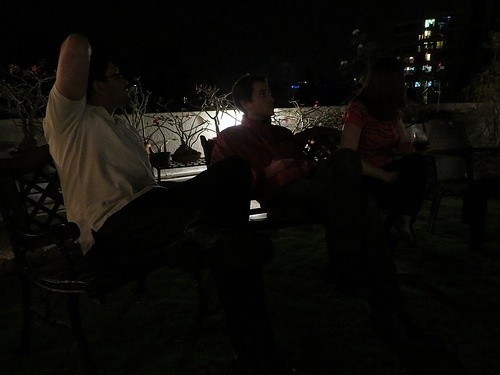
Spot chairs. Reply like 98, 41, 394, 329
201, 136, 215, 169
293, 125, 340, 174
0, 145, 209, 375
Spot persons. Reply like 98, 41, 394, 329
210, 75, 445, 345
43, 33, 298, 375
338, 56, 430, 245
